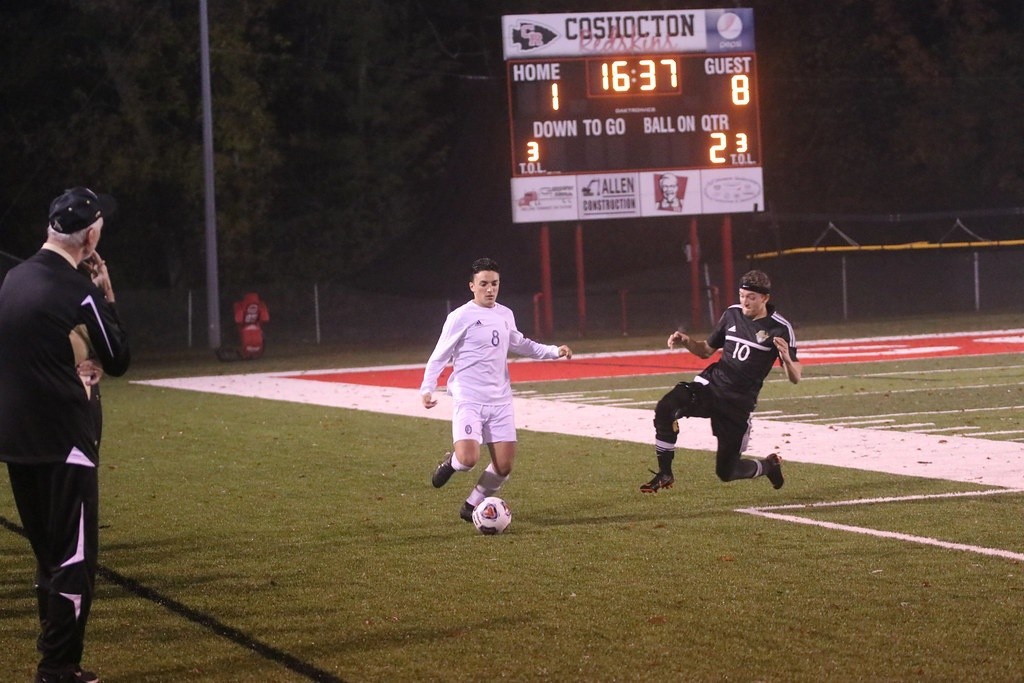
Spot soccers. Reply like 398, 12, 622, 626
471, 495, 513, 535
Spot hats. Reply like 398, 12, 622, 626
49, 186, 116, 234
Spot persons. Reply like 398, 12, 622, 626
1, 187, 131, 683
639, 269, 802, 494
419, 257, 573, 523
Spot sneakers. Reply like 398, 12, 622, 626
767, 454, 784, 490
639, 468, 674, 493
432, 452, 457, 488
459, 501, 476, 522
35, 668, 99, 683
